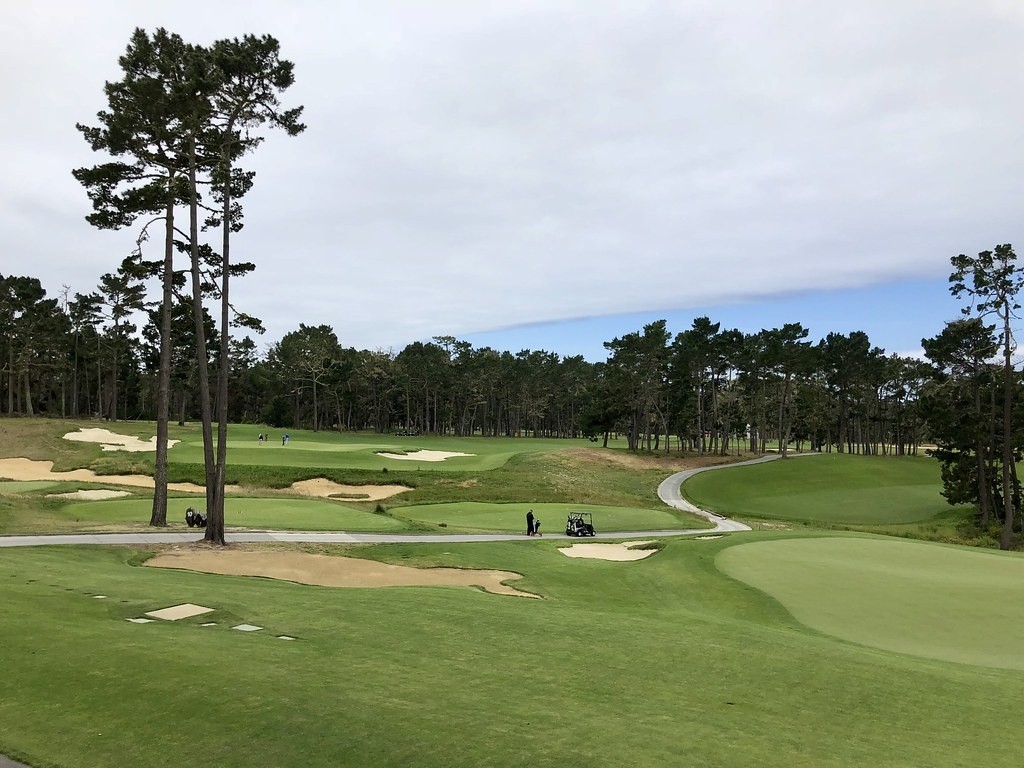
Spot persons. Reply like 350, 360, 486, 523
285, 434, 289, 445
526, 510, 534, 536
258, 433, 263, 445
